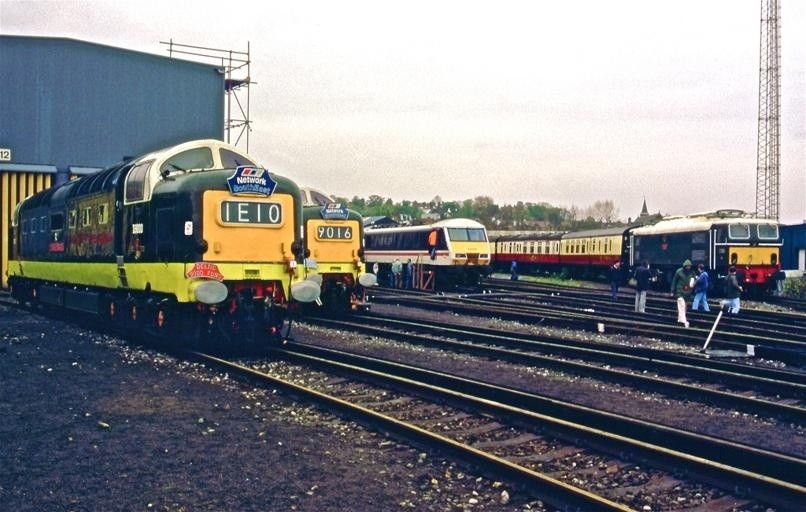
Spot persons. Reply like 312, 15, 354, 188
372, 260, 381, 285
428, 229, 438, 260
669, 259, 697, 328
608, 258, 622, 301
510, 258, 519, 281
391, 257, 402, 288
720, 267, 731, 309
723, 266, 745, 314
692, 264, 712, 311
633, 259, 651, 313
405, 258, 414, 288
